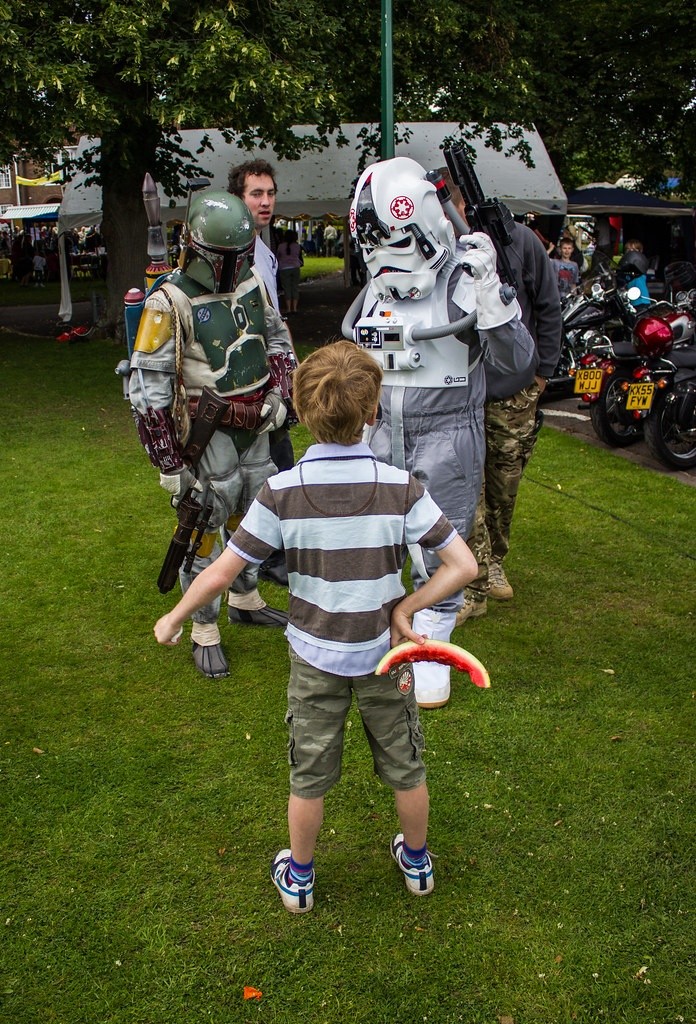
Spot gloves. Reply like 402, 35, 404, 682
459, 230, 523, 330
254, 385, 290, 436
160, 463, 203, 505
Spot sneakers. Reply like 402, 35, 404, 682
270, 848, 316, 914
487, 560, 515, 601
455, 595, 488, 628
389, 832, 438, 895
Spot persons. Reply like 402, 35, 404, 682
154, 340, 477, 912
514, 213, 652, 312
0, 224, 103, 288
128, 158, 562, 709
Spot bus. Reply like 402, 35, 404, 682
566, 214, 595, 232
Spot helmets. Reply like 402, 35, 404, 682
664, 261, 696, 291
617, 251, 649, 284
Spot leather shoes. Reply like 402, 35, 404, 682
259, 563, 291, 587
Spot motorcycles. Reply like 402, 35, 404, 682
538, 261, 696, 471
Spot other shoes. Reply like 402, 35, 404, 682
410, 608, 457, 709
32, 282, 39, 287
20, 284, 28, 288
40, 283, 45, 287
193, 639, 231, 678
227, 604, 290, 627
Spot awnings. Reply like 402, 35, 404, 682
1, 203, 61, 220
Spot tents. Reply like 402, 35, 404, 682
58, 122, 567, 322
566, 175, 696, 216
